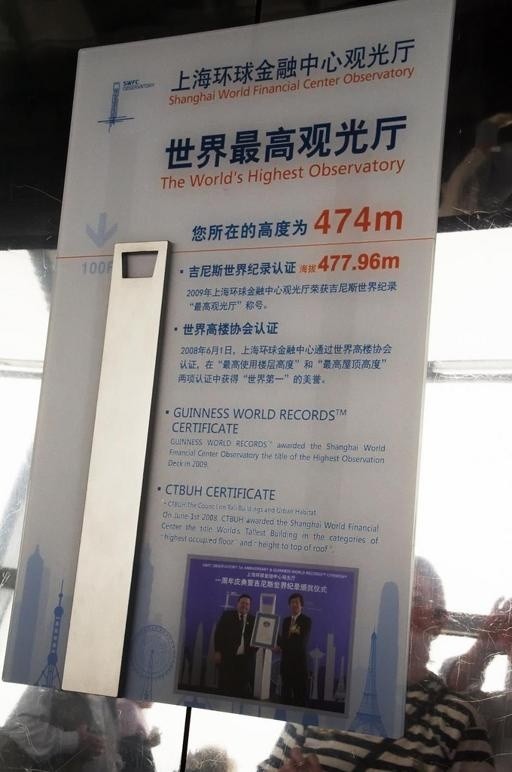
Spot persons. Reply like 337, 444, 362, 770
257, 554, 496, 772
214, 595, 256, 699
114, 696, 161, 772
1, 684, 126, 772
437, 594, 512, 772
186, 745, 237, 772
276, 593, 312, 706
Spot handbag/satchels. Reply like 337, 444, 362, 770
0, 693, 102, 772
119, 732, 154, 772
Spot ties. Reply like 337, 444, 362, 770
240, 615, 244, 637
